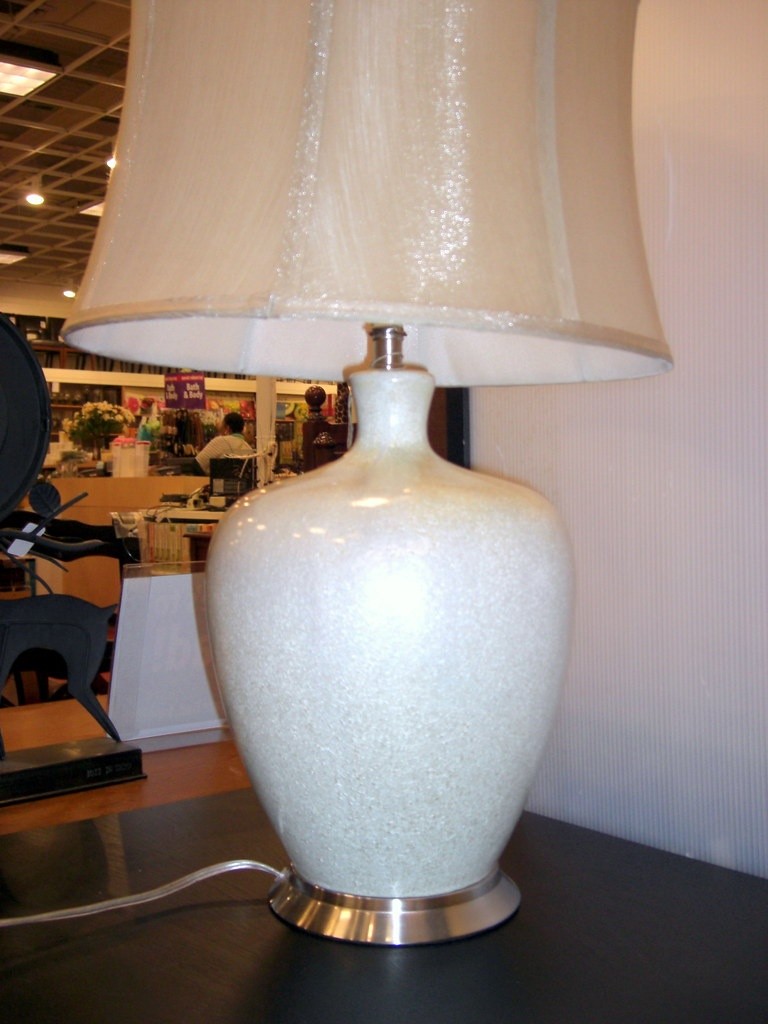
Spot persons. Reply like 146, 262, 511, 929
187, 412, 255, 477
138, 398, 178, 466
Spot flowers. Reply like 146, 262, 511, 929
63, 401, 135, 454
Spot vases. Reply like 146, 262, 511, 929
92, 439, 101, 461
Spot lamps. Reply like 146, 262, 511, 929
25, 175, 47, 207
62, 278, 77, 298
56, 0, 673, 948
